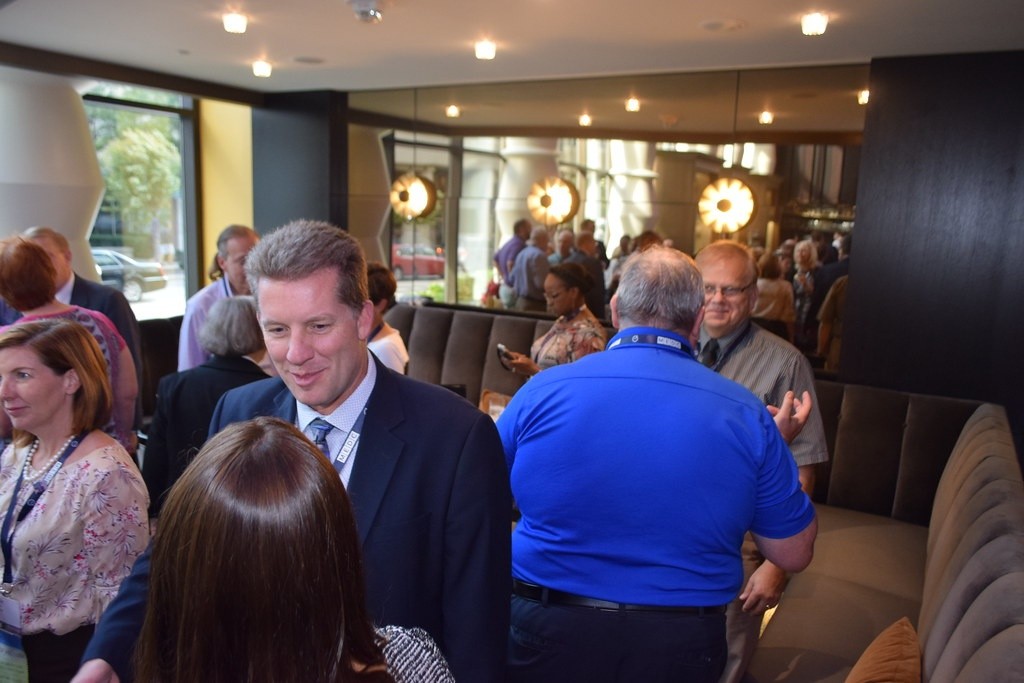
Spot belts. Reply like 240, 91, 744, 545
511, 578, 726, 615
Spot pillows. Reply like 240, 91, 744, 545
480, 389, 512, 413
845, 617, 922, 683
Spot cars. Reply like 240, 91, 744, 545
90, 250, 166, 302
391, 244, 445, 280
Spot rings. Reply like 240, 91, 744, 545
512, 368, 516, 372
766, 604, 770, 609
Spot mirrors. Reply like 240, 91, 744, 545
348, 63, 869, 368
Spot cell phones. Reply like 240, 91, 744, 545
498, 343, 517, 360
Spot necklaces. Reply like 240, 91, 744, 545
23, 435, 77, 482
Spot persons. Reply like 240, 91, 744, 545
681, 240, 827, 683
500, 263, 607, 380
142, 297, 275, 538
753, 231, 850, 380
138, 417, 397, 682
361, 263, 409, 376
177, 224, 262, 371
1, 318, 151, 683
491, 247, 819, 683
0, 226, 149, 469
69, 221, 510, 683
494, 220, 660, 316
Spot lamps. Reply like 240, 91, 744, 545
527, 177, 581, 226
390, 172, 437, 221
698, 178, 757, 234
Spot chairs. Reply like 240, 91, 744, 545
136, 316, 183, 446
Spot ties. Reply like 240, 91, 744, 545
309, 418, 334, 460
701, 339, 719, 366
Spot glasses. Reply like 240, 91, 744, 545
704, 281, 755, 296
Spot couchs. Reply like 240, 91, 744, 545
383, 303, 1024, 683
